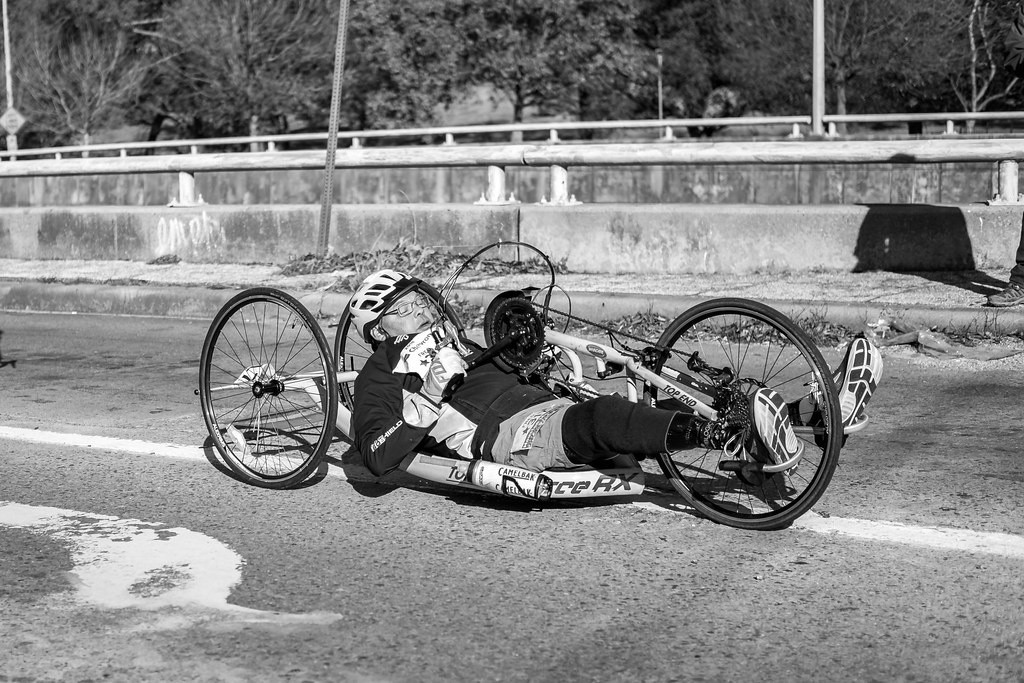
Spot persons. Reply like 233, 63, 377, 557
987, 211, 1024, 307
350, 268, 884, 479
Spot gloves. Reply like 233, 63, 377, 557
541, 344, 563, 372
423, 347, 469, 396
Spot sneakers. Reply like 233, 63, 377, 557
803, 337, 884, 450
724, 388, 804, 476
987, 283, 1024, 307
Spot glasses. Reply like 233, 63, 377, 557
384, 294, 430, 318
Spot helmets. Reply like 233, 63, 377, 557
348, 269, 424, 343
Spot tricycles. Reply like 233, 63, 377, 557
195, 240, 870, 530
